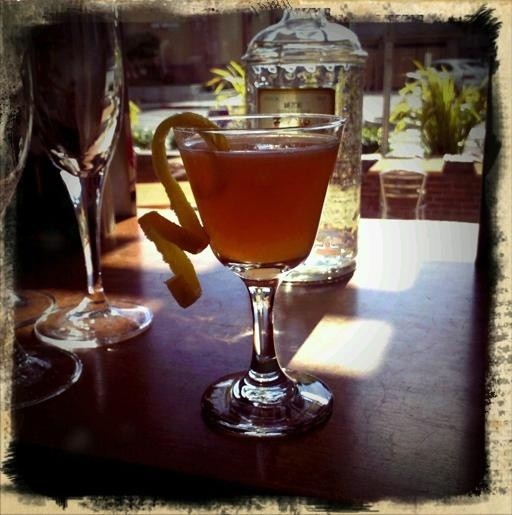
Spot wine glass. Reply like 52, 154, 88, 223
0, 29, 82, 412
30, 12, 153, 350
174, 114, 350, 434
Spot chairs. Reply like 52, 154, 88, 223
379, 169, 429, 222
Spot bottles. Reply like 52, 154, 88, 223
240, 6, 369, 287
